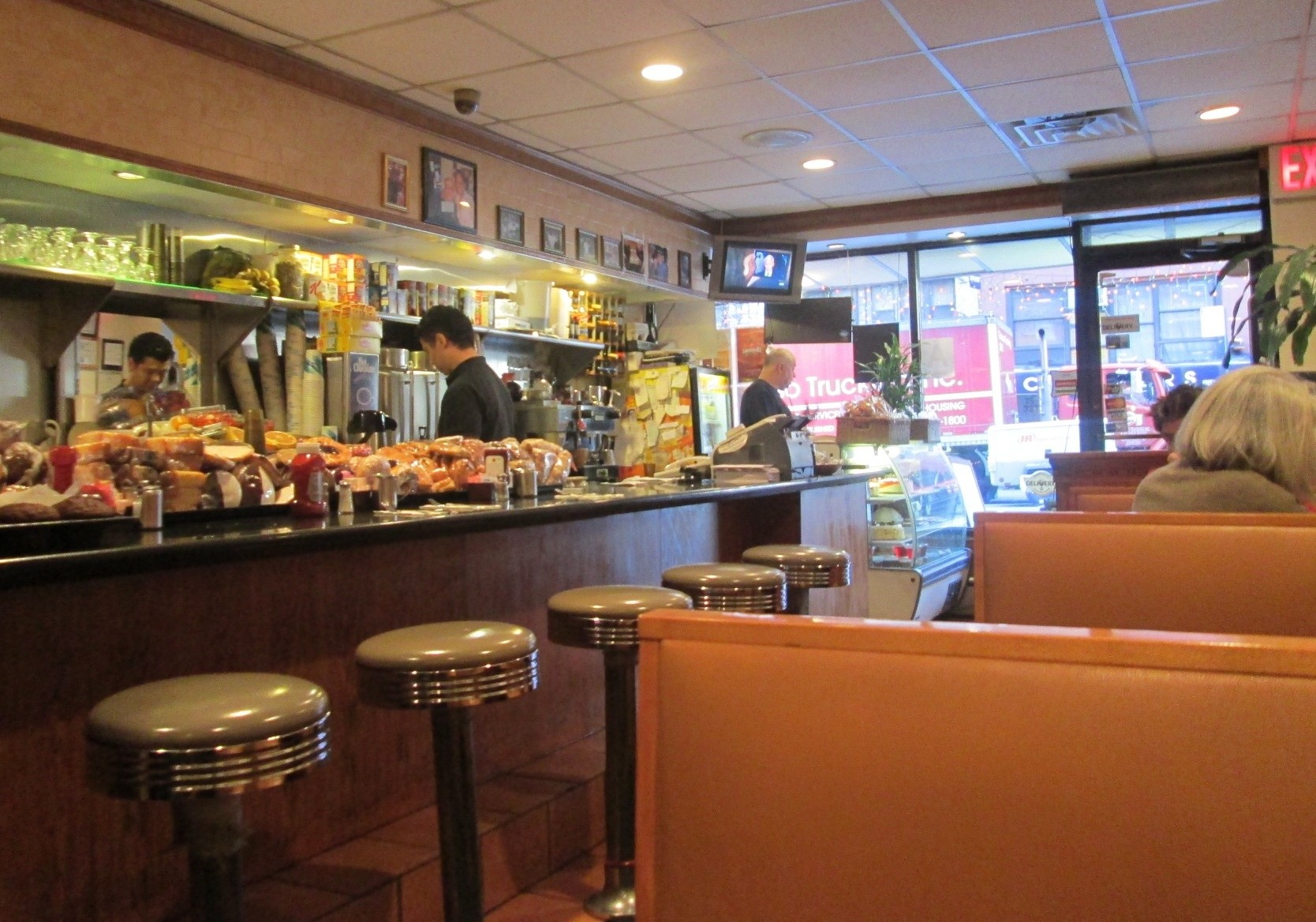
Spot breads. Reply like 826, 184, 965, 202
0, 417, 571, 522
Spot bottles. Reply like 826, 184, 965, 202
140, 485, 163, 531
274, 243, 304, 300
497, 473, 510, 502
337, 481, 354, 515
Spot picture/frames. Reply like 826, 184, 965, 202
622, 233, 646, 278
540, 217, 567, 258
600, 235, 622, 272
80, 311, 100, 335
495, 205, 525, 248
647, 242, 669, 284
421, 146, 479, 236
576, 228, 599, 266
101, 339, 125, 371
677, 250, 692, 290
380, 152, 409, 214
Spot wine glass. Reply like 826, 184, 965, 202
0, 217, 158, 283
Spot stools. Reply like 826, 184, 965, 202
661, 562, 788, 615
354, 620, 540, 922
84, 670, 332, 922
742, 543, 852, 615
546, 584, 696, 922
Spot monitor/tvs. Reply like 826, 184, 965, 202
708, 235, 808, 303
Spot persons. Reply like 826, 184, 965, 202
105, 332, 174, 407
416, 305, 516, 442
650, 251, 666, 278
758, 255, 777, 278
1134, 364, 1316, 512
1150, 384, 1204, 452
740, 348, 817, 432
429, 169, 474, 228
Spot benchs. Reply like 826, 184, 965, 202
633, 453, 1316, 922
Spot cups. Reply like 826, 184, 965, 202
303, 348, 325, 437
514, 469, 538, 499
134, 222, 184, 287
589, 384, 601, 405
377, 475, 398, 512
244, 409, 266, 455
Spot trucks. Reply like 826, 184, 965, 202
772, 317, 1172, 503
